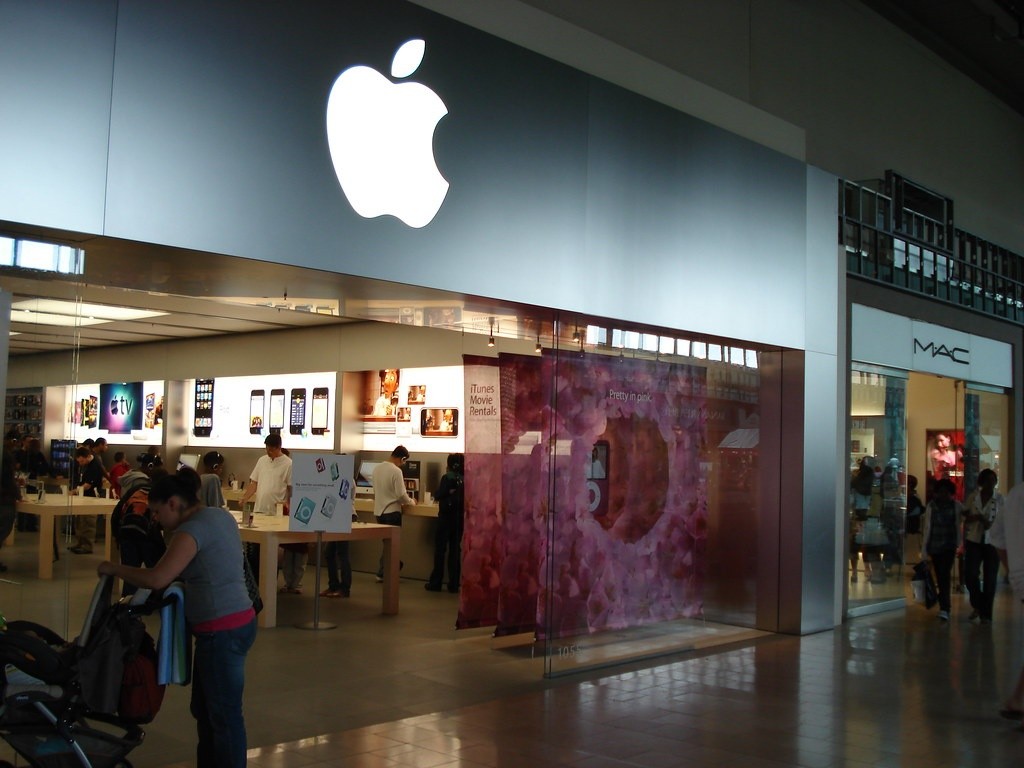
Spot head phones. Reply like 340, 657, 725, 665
12, 432, 18, 444
148, 455, 157, 471
401, 452, 409, 464
213, 453, 220, 470
228, 473, 236, 487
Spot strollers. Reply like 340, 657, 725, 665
0, 574, 178, 768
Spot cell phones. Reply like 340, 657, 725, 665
71, 396, 97, 428
194, 378, 215, 437
37, 491, 43, 500
249, 388, 329, 435
145, 393, 156, 429
420, 408, 458, 438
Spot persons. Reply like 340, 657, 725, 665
109, 445, 225, 617
985, 482, 1024, 720
239, 434, 309, 595
848, 453, 926, 583
931, 431, 962, 482
97, 466, 258, 768
373, 446, 416, 583
319, 453, 358, 598
154, 404, 163, 425
424, 453, 464, 594
921, 480, 968, 620
958, 468, 1005, 626
0, 434, 108, 573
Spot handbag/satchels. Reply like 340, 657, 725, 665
911, 561, 939, 610
243, 559, 263, 615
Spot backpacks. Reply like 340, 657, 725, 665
115, 482, 160, 556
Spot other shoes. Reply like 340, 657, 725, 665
937, 611, 953, 620
980, 617, 991, 624
969, 609, 981, 618
425, 582, 441, 591
286, 587, 304, 593
319, 589, 346, 598
71, 545, 93, 554
376, 576, 384, 582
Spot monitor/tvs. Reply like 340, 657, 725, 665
100, 382, 143, 434
176, 454, 201, 473
355, 460, 384, 494
